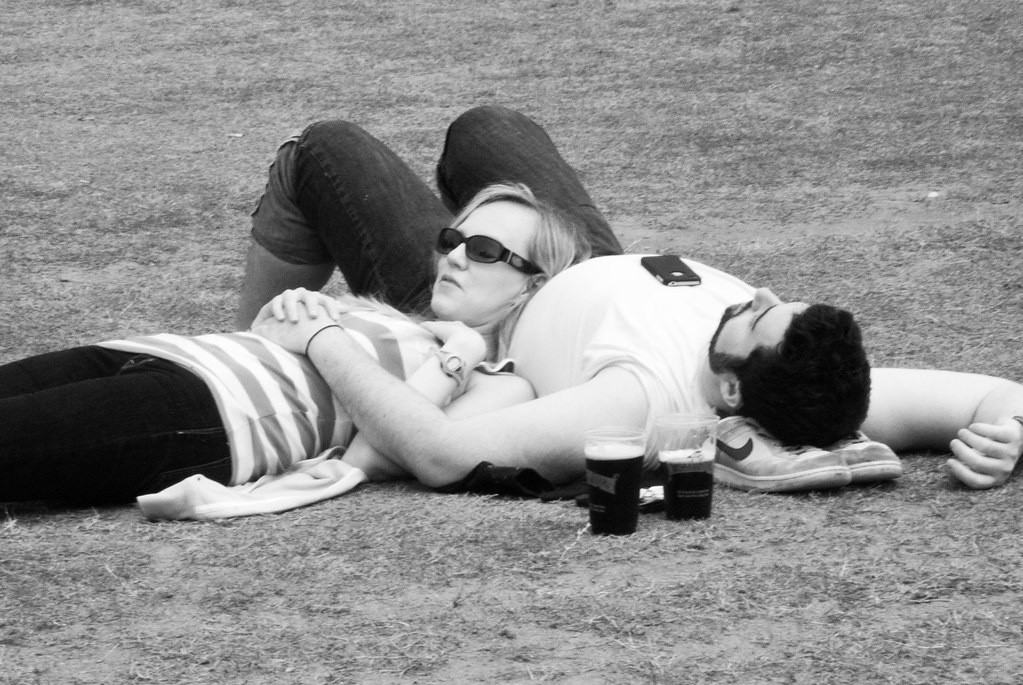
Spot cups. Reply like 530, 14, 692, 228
656, 411, 720, 521
581, 426, 649, 535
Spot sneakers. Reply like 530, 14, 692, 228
713, 415, 852, 492
823, 428, 902, 483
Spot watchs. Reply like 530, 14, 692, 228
434, 345, 467, 383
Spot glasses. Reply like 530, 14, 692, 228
434, 228, 542, 276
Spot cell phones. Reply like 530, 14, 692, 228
641, 255, 700, 287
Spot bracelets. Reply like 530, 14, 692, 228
1013, 415, 1023, 426
306, 325, 344, 356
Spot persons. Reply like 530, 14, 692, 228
236, 104, 1023, 490
0, 185, 592, 523
